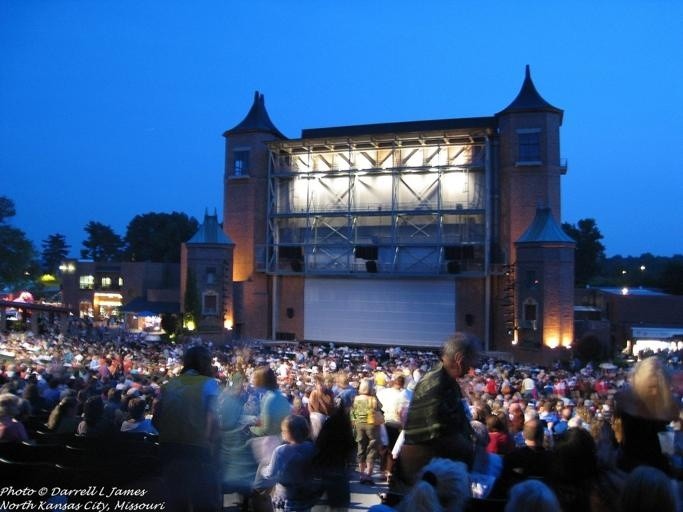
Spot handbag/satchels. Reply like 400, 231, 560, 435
367, 409, 384, 425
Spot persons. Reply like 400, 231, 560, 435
0, 282, 683, 512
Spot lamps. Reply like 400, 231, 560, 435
291, 261, 302, 270
446, 262, 459, 274
366, 262, 379, 273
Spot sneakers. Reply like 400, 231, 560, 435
360, 476, 375, 485
